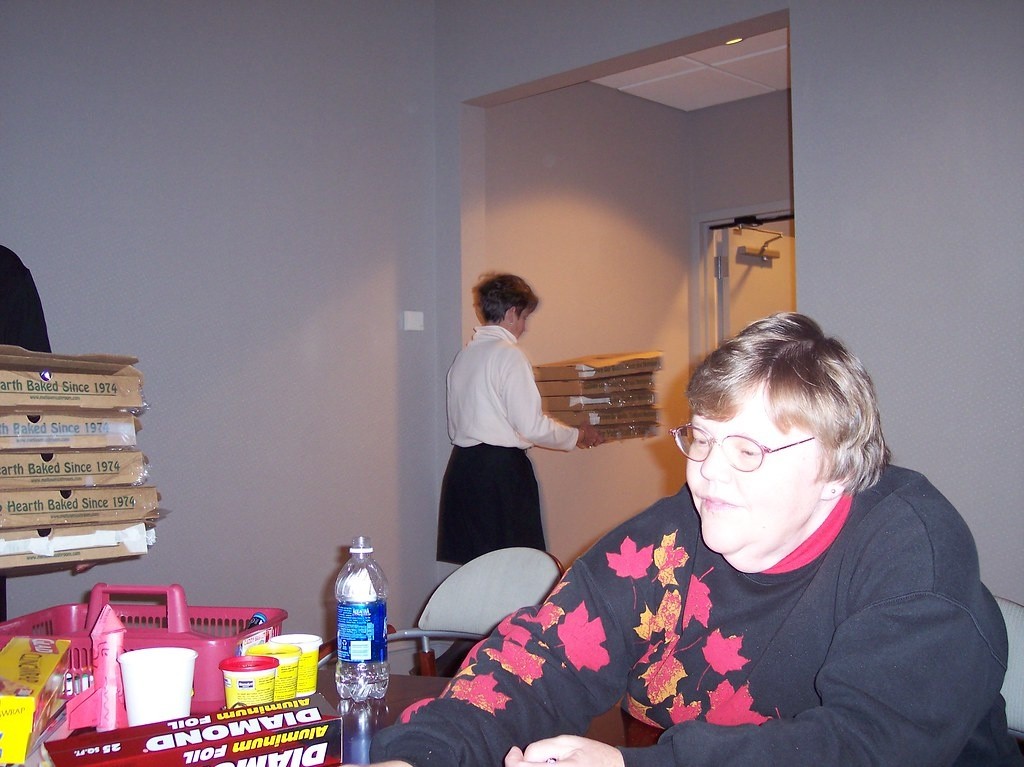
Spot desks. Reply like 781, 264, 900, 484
317, 669, 667, 750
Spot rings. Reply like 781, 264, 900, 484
589, 445, 592, 448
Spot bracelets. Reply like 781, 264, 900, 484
580, 428, 586, 443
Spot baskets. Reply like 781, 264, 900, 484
2, 583, 290, 716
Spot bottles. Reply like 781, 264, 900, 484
335, 537, 390, 703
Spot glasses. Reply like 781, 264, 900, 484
668, 422, 814, 472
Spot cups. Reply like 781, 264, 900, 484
219, 633, 323, 710
116, 646, 199, 727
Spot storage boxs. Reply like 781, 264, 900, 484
533, 352, 663, 448
0, 343, 160, 578
317, 546, 563, 676
41, 692, 344, 766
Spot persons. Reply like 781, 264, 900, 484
0, 243, 101, 620
342, 311, 1010, 767
436, 274, 606, 564
